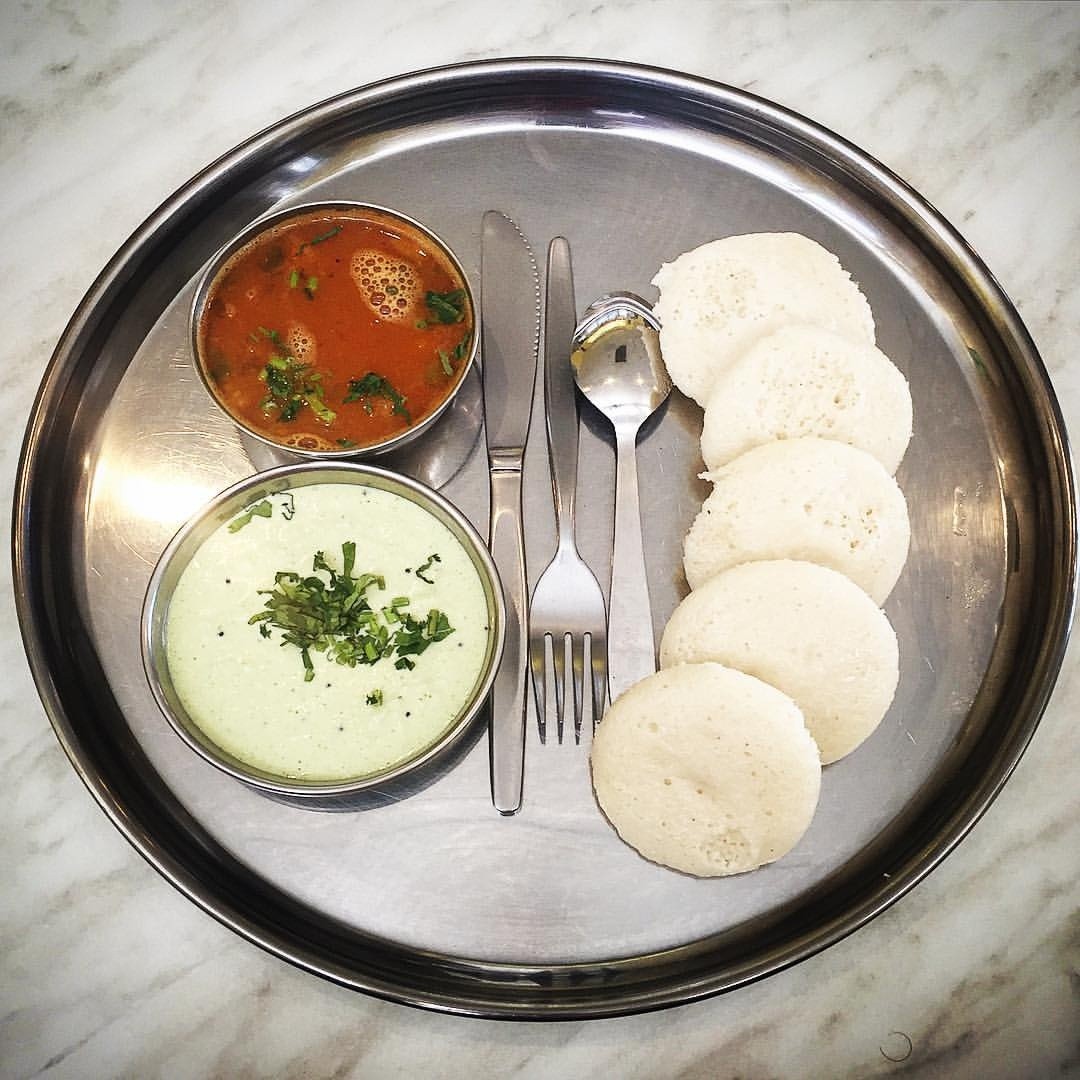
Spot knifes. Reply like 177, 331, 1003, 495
480, 209, 541, 814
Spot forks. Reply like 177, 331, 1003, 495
529, 237, 609, 726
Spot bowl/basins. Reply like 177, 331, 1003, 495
188, 202, 480, 462
141, 463, 509, 798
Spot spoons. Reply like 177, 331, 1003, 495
568, 292, 673, 704
